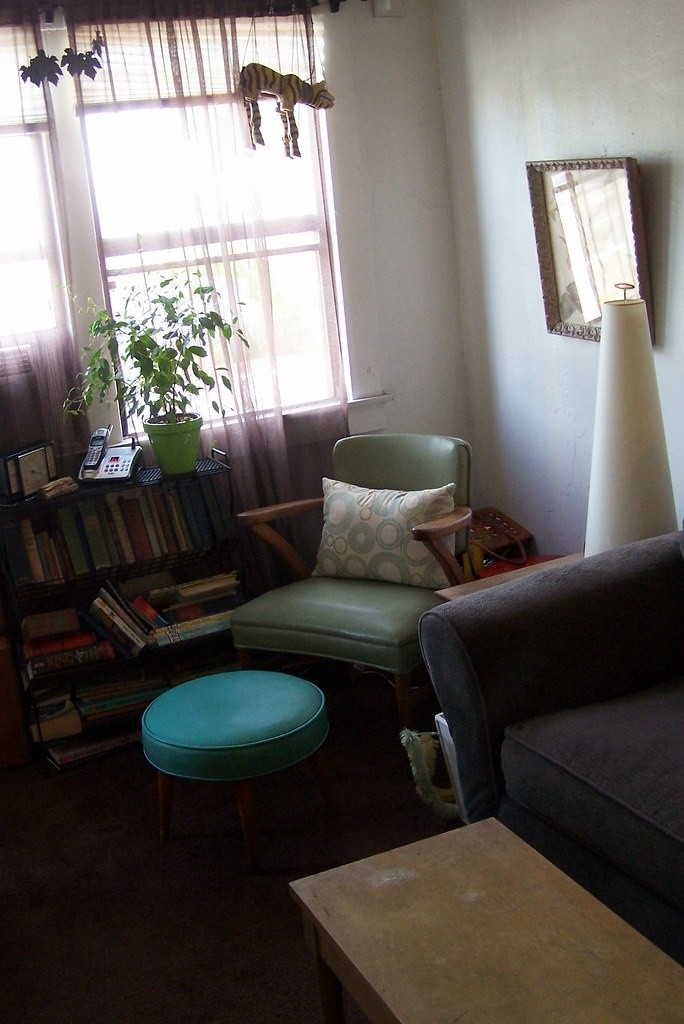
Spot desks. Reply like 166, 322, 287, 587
287, 817, 684, 1024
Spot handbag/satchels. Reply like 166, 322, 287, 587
468, 508, 531, 565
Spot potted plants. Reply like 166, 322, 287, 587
52, 251, 250, 476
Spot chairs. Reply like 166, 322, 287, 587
233, 433, 474, 743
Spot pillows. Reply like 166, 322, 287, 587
310, 476, 456, 589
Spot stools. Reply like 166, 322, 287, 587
141, 669, 331, 866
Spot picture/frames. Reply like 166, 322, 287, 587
525, 158, 657, 347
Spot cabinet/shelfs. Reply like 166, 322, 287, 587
0, 447, 259, 780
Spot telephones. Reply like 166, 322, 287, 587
77, 424, 143, 483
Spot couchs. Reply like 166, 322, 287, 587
418, 531, 684, 969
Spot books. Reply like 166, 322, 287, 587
15, 559, 242, 682
18, 465, 231, 589
29, 669, 198, 772
